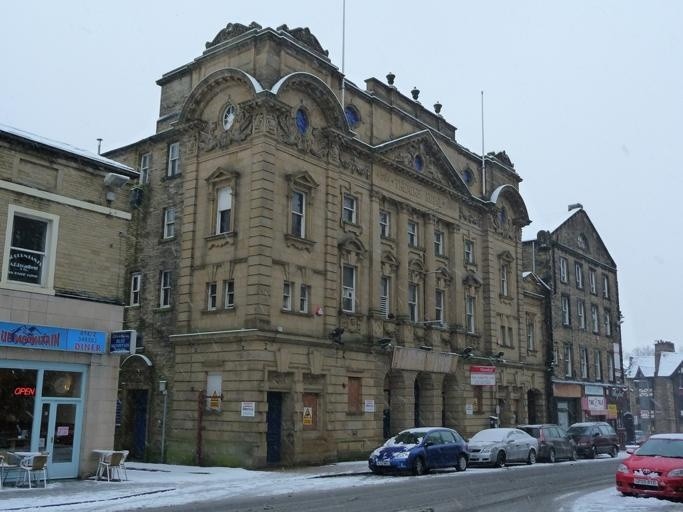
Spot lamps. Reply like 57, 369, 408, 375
103, 173, 130, 201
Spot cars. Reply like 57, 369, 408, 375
616, 431, 683, 501
466, 419, 623, 468
367, 425, 471, 475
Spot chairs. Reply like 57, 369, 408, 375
88, 450, 129, 483
0, 451, 50, 489
